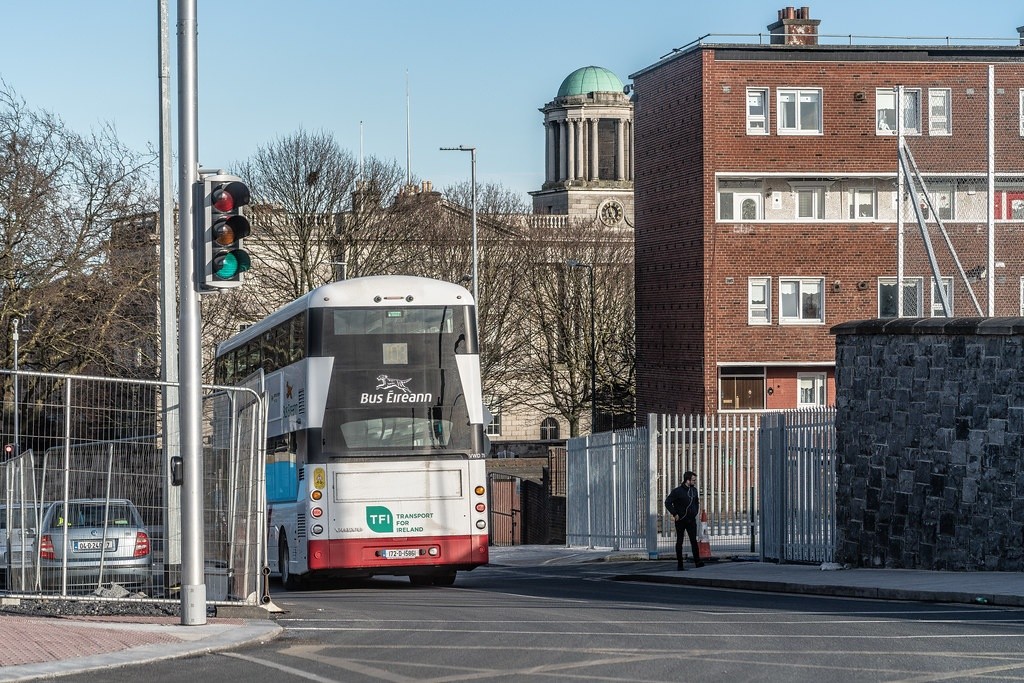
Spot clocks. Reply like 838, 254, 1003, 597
600, 200, 624, 227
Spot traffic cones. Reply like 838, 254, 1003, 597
687, 511, 719, 563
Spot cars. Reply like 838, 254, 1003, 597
1, 502, 53, 591
31, 497, 154, 597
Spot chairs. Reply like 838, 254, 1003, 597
107, 510, 127, 525
60, 510, 79, 527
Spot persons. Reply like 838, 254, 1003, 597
665, 471, 706, 571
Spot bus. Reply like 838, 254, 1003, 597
213, 275, 491, 593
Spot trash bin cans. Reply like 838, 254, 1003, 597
203, 559, 229, 609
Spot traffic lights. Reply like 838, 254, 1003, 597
197, 165, 252, 296
